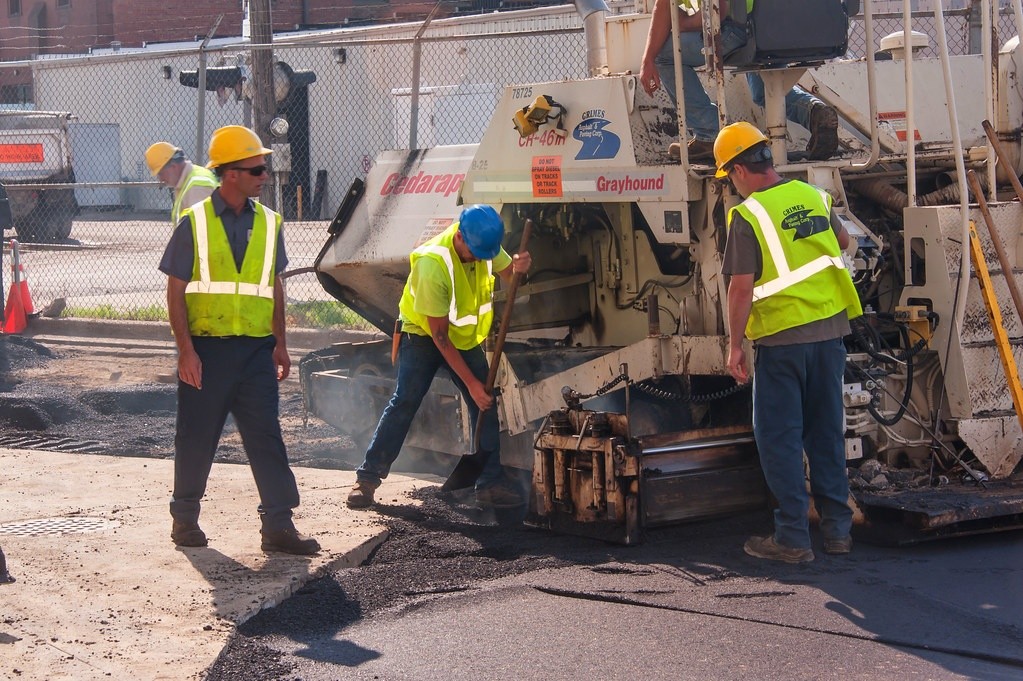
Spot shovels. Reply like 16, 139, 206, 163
441, 219, 532, 491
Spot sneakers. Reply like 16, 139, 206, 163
823, 533, 853, 553
171, 516, 207, 547
806, 102, 838, 160
260, 520, 320, 554
346, 479, 375, 507
668, 137, 714, 160
475, 484, 526, 509
743, 535, 815, 563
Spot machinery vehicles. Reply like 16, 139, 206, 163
294, 1, 1022, 548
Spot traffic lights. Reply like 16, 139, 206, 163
178, 64, 243, 104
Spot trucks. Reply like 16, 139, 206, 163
0, 108, 80, 245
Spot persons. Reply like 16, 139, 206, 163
158, 125, 321, 556
714, 121, 863, 563
347, 205, 532, 507
640, 0, 839, 160
146, 141, 221, 382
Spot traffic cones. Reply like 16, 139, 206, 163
7, 239, 43, 318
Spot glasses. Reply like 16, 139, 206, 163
222, 165, 268, 176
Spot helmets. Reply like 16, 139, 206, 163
205, 124, 274, 168
713, 122, 769, 179
460, 204, 504, 260
144, 141, 178, 176
